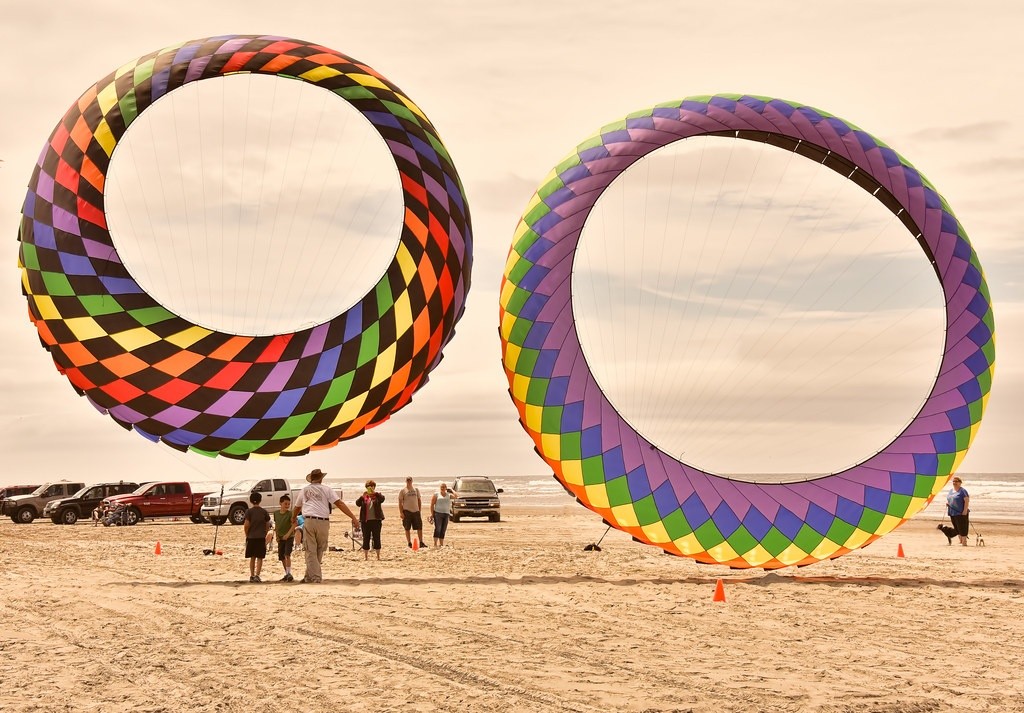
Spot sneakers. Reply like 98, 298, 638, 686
408, 542, 412, 548
279, 573, 293, 582
420, 541, 427, 547
250, 575, 261, 583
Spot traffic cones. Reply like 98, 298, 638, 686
896, 542, 905, 558
712, 578, 726, 601
412, 539, 420, 551
154, 541, 162, 555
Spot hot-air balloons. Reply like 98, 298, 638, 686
16, 32, 472, 460
491, 94, 1003, 570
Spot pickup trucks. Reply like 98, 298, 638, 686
100, 481, 223, 523
201, 476, 344, 525
1, 482, 106, 524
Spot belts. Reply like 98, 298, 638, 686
305, 516, 329, 520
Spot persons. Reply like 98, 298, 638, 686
243, 491, 269, 587
94, 500, 108, 527
354, 480, 388, 563
102, 501, 134, 528
946, 476, 971, 547
273, 495, 298, 584
290, 468, 360, 585
397, 475, 430, 552
292, 514, 305, 553
428, 482, 458, 550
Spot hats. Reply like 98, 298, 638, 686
306, 469, 327, 483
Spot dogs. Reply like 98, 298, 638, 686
936, 524, 969, 546
976, 533, 984, 547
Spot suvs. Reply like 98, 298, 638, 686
42, 480, 142, 525
447, 474, 505, 523
